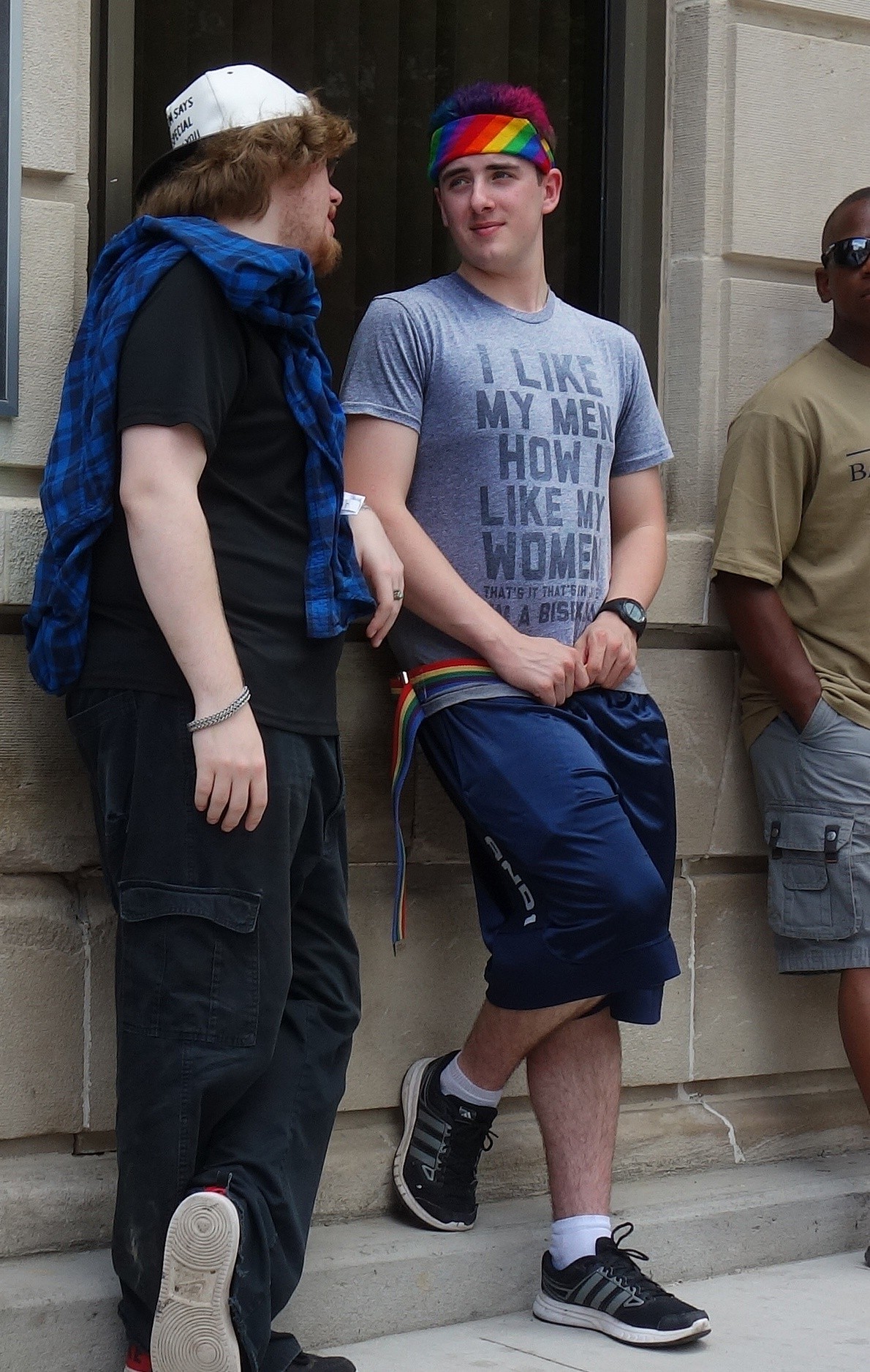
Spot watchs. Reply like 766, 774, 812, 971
590, 596, 648, 643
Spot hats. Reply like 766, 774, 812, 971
132, 65, 316, 205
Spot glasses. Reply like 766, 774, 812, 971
820, 237, 870, 268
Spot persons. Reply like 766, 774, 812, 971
25, 65, 402, 1372
710, 183, 870, 1272
338, 87, 714, 1348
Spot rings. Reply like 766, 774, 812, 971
393, 590, 402, 600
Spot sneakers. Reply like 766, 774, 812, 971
532, 1221, 712, 1346
392, 1049, 499, 1232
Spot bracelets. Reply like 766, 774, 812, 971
187, 684, 251, 733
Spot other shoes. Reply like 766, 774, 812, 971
124, 1343, 356, 1372
150, 1191, 245, 1372
